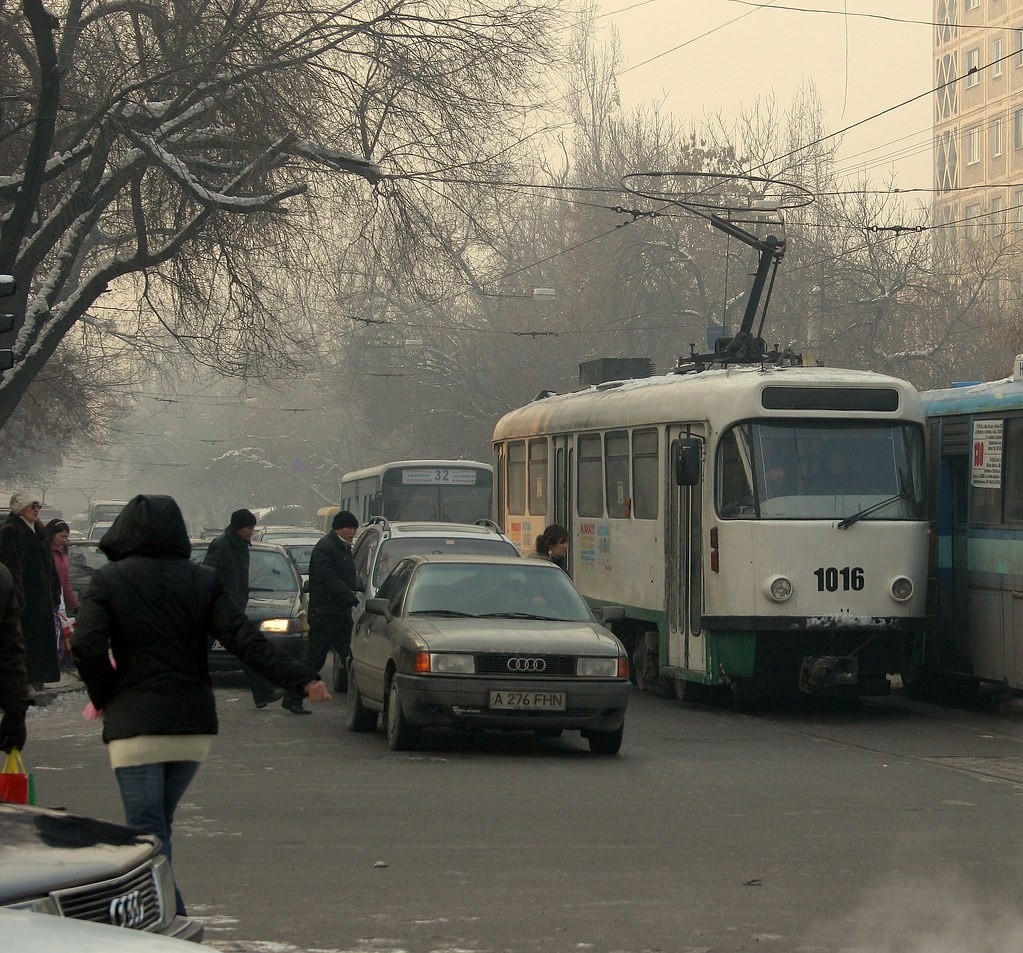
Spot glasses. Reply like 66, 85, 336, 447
28, 505, 42, 511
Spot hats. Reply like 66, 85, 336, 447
231, 509, 257, 530
332, 511, 358, 530
10, 491, 42, 516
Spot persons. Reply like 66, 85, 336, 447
0, 489, 81, 755
476, 568, 545, 615
528, 524, 572, 577
70, 494, 333, 916
740, 445, 877, 508
281, 510, 366, 715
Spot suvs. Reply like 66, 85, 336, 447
332, 515, 531, 692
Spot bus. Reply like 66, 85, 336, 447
88, 499, 130, 534
902, 353, 1023, 703
307, 506, 340, 535
491, 172, 936, 715
340, 459, 492, 541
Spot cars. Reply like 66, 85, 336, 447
1, 803, 203, 942
345, 554, 630, 755
65, 521, 329, 673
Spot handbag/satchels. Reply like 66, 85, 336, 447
0, 746, 36, 805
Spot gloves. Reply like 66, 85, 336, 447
343, 594, 360, 608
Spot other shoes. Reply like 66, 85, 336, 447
256, 691, 285, 708
282, 700, 312, 714
